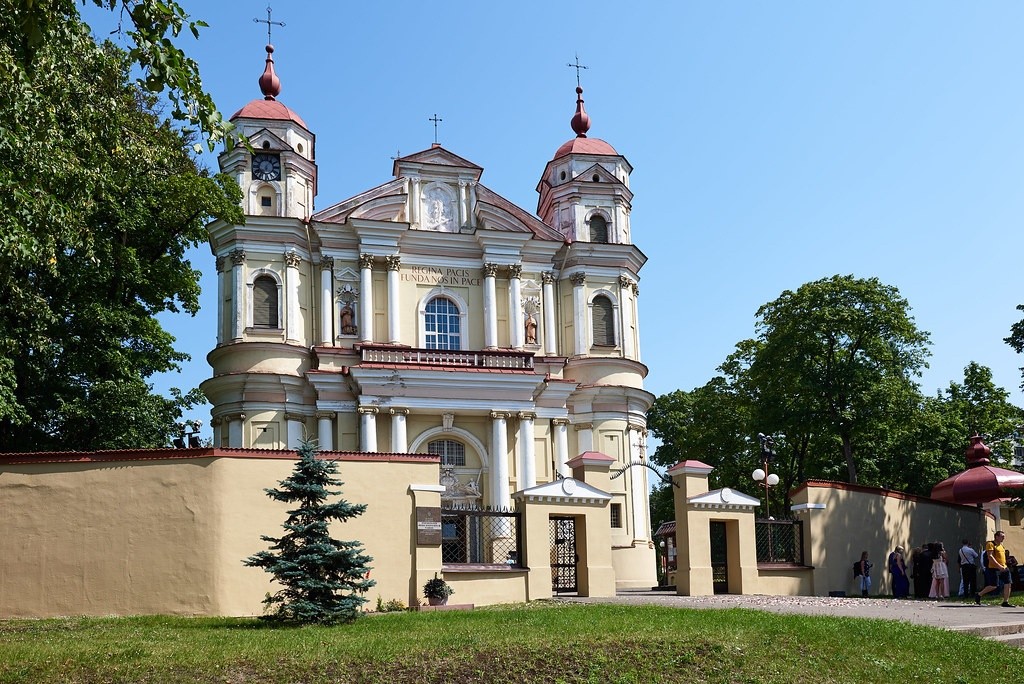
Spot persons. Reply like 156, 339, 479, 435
888, 541, 949, 600
957, 540, 1019, 601
524, 313, 537, 344
974, 531, 1017, 608
429, 187, 447, 233
858, 551, 873, 598
451, 474, 478, 495
339, 301, 358, 335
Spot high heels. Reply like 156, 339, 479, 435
936, 595, 940, 601
940, 595, 945, 601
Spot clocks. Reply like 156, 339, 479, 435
251, 152, 280, 182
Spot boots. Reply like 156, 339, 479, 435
862, 591, 868, 597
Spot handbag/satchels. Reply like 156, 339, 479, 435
969, 566, 977, 575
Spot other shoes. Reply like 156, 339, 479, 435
1001, 602, 1015, 607
975, 592, 982, 605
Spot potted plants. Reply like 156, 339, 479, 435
423, 578, 453, 605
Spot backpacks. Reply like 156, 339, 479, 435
853, 560, 863, 580
983, 541, 996, 570
